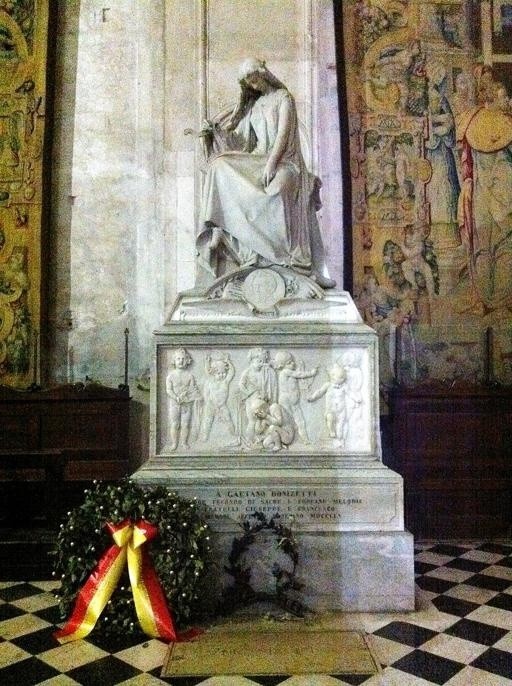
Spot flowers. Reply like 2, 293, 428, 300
49, 472, 216, 642
216, 507, 315, 617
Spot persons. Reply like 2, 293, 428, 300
160, 344, 352, 453
190, 54, 337, 289
5, 253, 30, 294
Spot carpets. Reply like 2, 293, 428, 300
157, 625, 382, 680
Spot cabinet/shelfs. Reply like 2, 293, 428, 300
0, 387, 130, 579
383, 381, 510, 540
